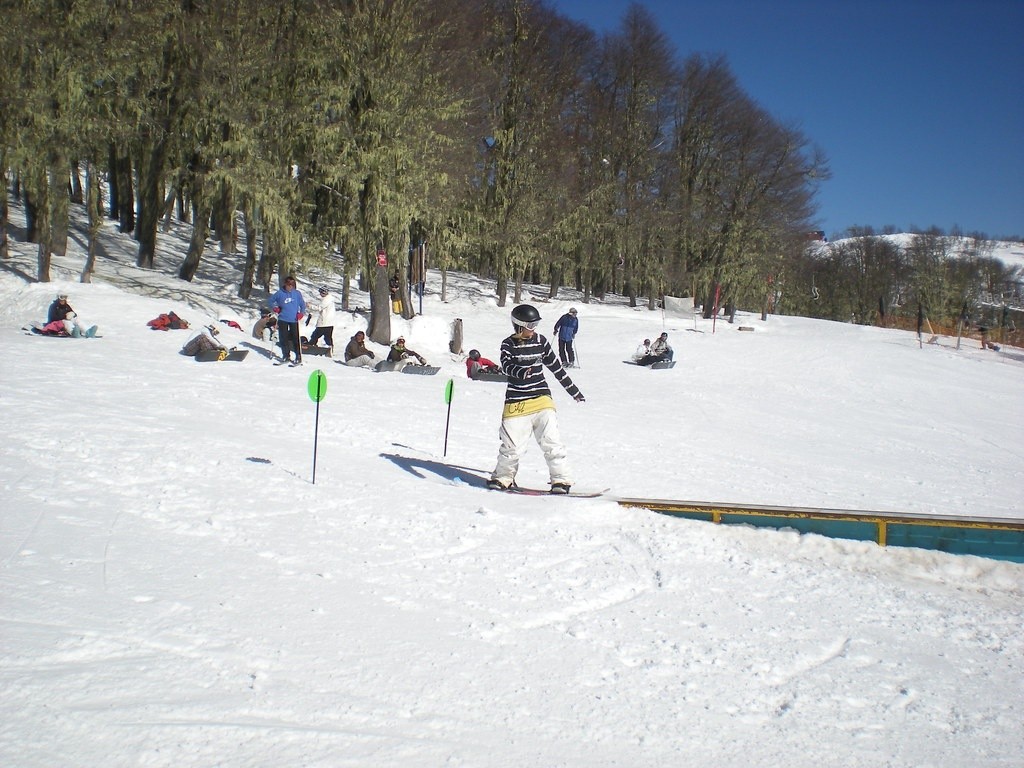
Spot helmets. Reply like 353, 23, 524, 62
511, 304, 541, 322
56, 290, 69, 301
568, 307, 578, 313
469, 349, 481, 361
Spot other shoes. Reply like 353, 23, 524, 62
72, 326, 82, 338
292, 359, 302, 366
281, 355, 290, 363
85, 325, 97, 337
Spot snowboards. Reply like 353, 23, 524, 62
402, 364, 442, 376
374, 360, 406, 373
487, 478, 611, 498
472, 373, 509, 383
196, 349, 249, 362
652, 361, 676, 369
301, 343, 330, 355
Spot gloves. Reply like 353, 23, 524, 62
367, 351, 375, 359
571, 333, 575, 339
273, 306, 281, 313
420, 357, 427, 365
402, 354, 409, 359
553, 329, 558, 335
296, 313, 304, 320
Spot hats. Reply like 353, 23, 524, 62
397, 336, 405, 343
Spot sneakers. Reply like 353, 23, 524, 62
486, 480, 507, 490
562, 361, 568, 367
550, 484, 570, 494
567, 361, 574, 368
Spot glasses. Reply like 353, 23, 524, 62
286, 281, 295, 286
511, 316, 540, 331
59, 295, 68, 301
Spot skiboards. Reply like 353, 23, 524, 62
273, 360, 304, 367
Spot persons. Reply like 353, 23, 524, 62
48, 290, 98, 338
466, 349, 502, 380
633, 333, 673, 365
182, 321, 237, 357
269, 277, 306, 364
554, 308, 578, 368
345, 331, 427, 371
389, 270, 403, 314
252, 309, 279, 344
978, 326, 1000, 351
307, 285, 335, 354
489, 305, 586, 494
